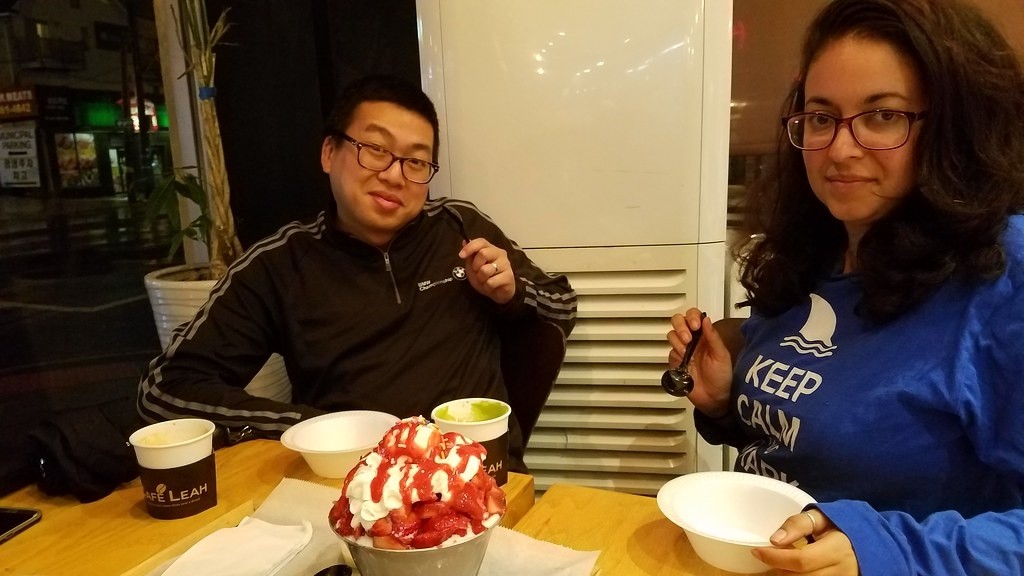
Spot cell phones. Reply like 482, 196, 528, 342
0, 506, 44, 547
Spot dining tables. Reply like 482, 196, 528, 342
495, 479, 817, 575
0, 435, 535, 576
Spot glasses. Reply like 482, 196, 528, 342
781, 109, 929, 151
340, 133, 440, 184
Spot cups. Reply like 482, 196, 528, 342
130, 418, 217, 519
430, 397, 512, 494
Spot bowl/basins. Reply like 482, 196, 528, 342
327, 501, 509, 576
280, 409, 402, 481
656, 471, 821, 573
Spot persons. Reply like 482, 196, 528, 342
662, 0, 1024, 576
135, 79, 581, 475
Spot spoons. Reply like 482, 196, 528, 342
660, 311, 708, 397
444, 204, 472, 246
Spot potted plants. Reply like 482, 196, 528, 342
145, 1, 296, 409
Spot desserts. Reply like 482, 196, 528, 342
328, 414, 506, 551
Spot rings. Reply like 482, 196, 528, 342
803, 512, 816, 536
491, 262, 498, 275
